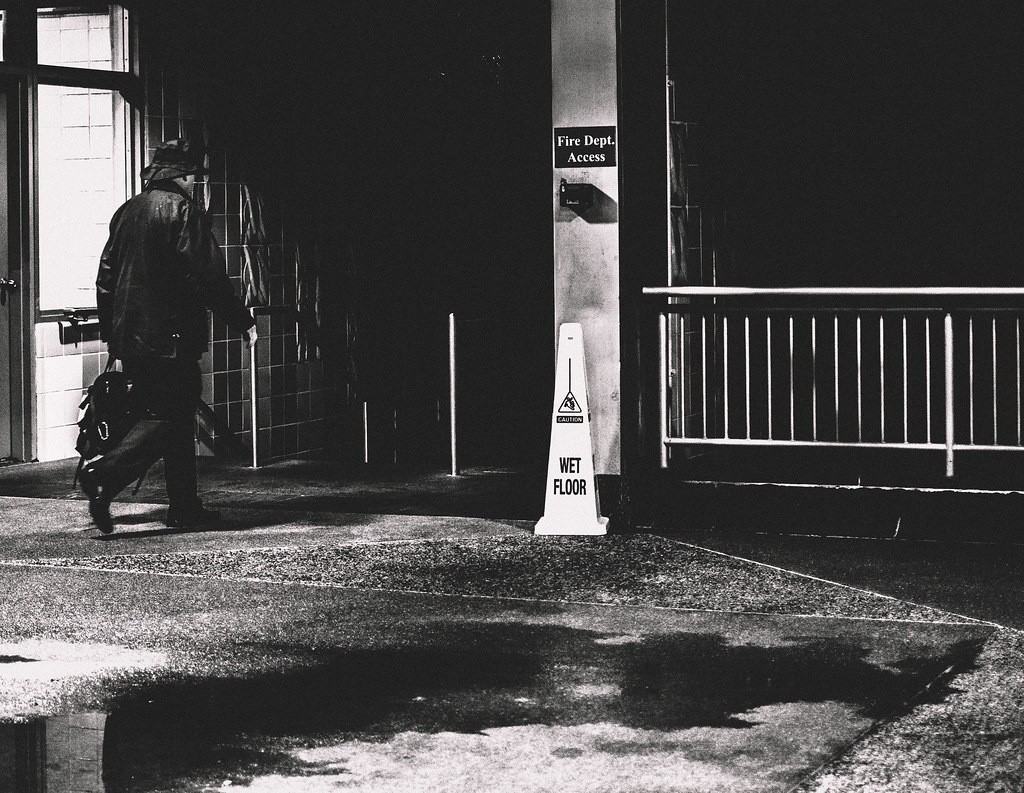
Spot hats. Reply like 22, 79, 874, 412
139, 139, 205, 182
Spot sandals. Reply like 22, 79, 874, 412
169, 508, 220, 529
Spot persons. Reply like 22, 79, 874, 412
80, 138, 258, 533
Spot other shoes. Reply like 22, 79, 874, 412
79, 467, 114, 533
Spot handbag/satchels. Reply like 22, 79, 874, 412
75, 356, 140, 459
